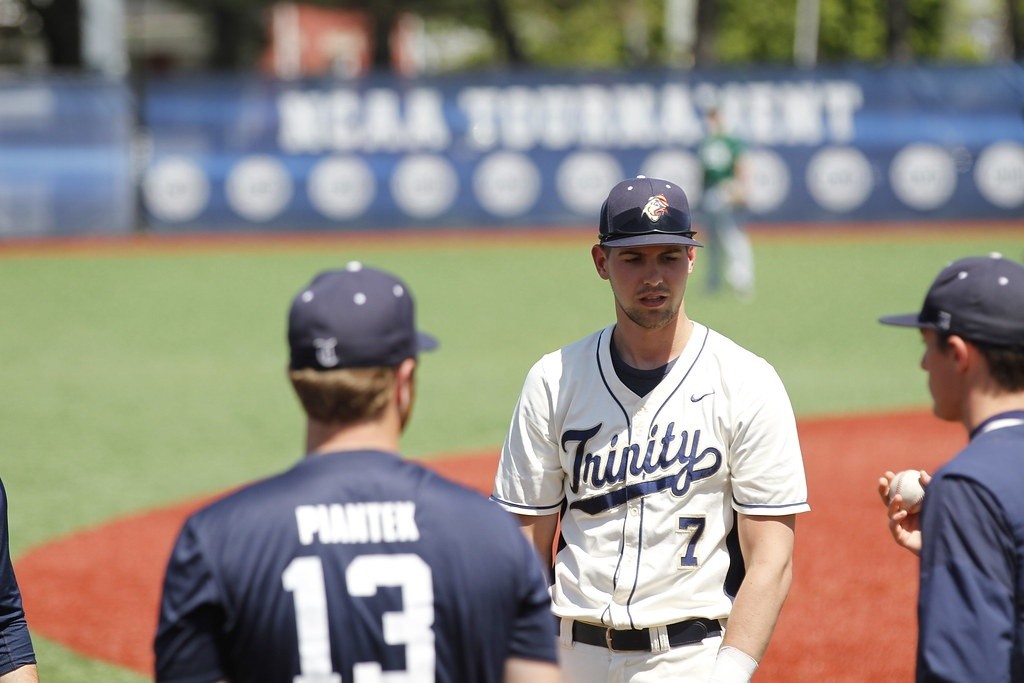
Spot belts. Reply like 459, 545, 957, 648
555, 616, 721, 650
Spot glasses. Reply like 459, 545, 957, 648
600, 206, 697, 240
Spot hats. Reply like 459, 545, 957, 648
289, 262, 439, 371
878, 257, 1024, 347
602, 174, 703, 247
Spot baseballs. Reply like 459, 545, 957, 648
887, 470, 927, 514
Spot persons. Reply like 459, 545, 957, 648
692, 98, 754, 287
488, 174, 811, 683
878, 254, 1024, 682
152, 261, 562, 682
0, 479, 40, 683
